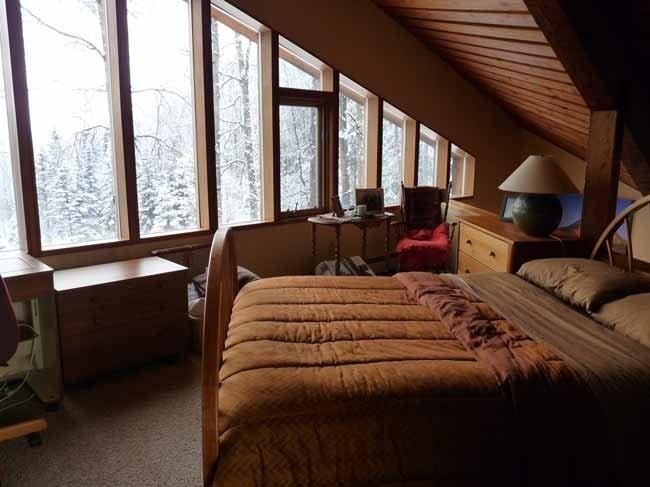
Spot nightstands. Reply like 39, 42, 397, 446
455, 213, 585, 281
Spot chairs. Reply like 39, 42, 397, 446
0, 275, 49, 450
400, 182, 452, 273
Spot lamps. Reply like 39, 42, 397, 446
497, 153, 582, 239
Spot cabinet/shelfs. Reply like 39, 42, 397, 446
52, 254, 191, 386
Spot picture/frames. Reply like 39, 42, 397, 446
355, 188, 385, 216
498, 192, 521, 222
329, 194, 345, 217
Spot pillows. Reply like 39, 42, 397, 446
516, 257, 650, 314
591, 291, 650, 351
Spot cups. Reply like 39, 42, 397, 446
357, 204, 367, 215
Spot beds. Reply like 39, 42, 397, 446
200, 193, 650, 486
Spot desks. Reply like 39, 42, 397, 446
308, 207, 395, 276
0, 252, 65, 408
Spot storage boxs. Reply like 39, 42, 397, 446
188, 313, 203, 356
